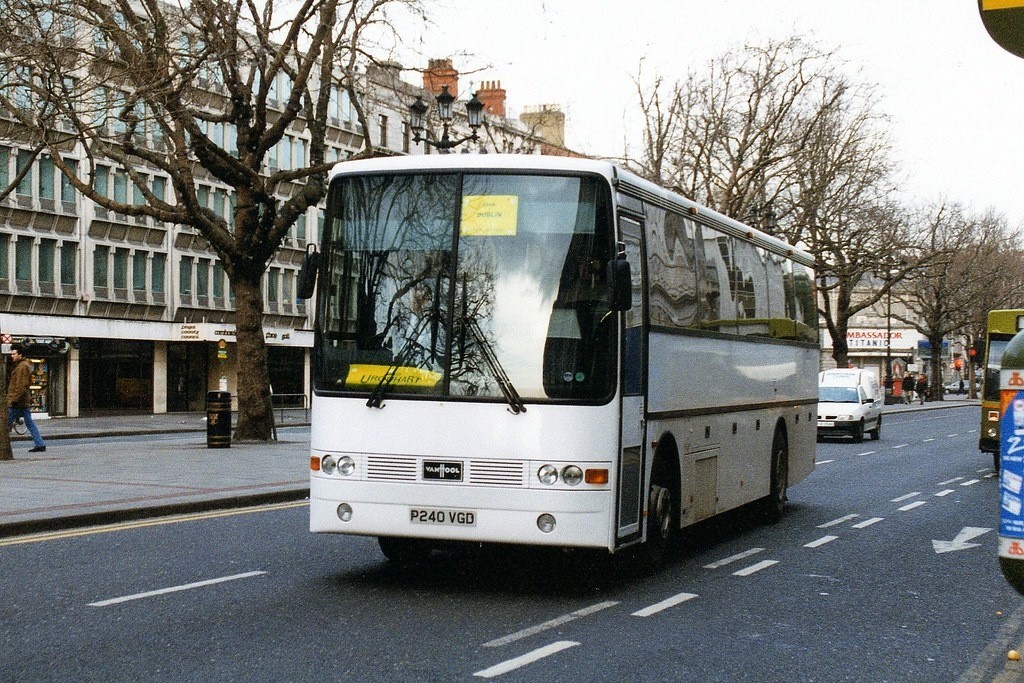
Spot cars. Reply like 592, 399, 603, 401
944, 380, 981, 395
813, 367, 882, 443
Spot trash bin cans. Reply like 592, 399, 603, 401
206, 390, 233, 449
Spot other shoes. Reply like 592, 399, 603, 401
28, 445, 46, 452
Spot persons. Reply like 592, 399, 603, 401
956, 379, 966, 396
6, 344, 46, 452
914, 374, 928, 404
900, 372, 915, 405
371, 278, 449, 356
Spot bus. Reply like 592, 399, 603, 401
296, 152, 824, 574
978, 309, 1024, 472
